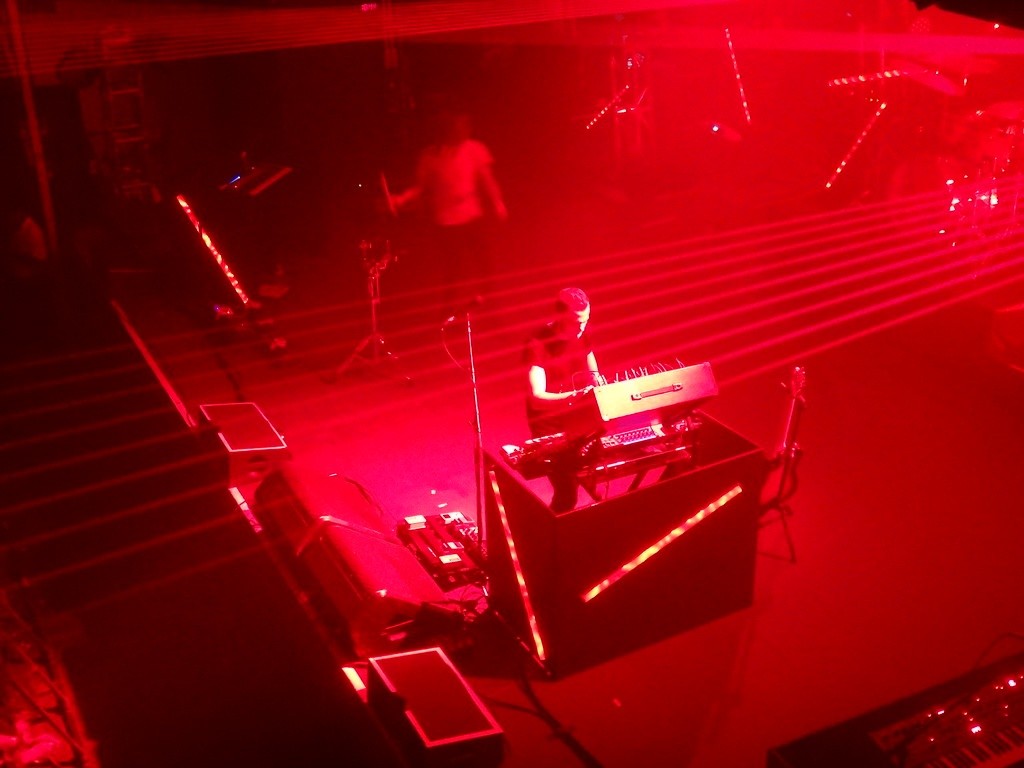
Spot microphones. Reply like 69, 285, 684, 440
789, 365, 806, 399
447, 295, 485, 321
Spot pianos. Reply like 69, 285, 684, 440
475, 360, 768, 682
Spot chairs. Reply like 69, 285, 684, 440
756, 364, 810, 564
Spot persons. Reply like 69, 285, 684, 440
0, 195, 48, 357
522, 287, 605, 514
389, 102, 509, 324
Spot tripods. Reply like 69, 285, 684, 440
337, 269, 411, 380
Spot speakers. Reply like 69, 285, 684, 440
366, 647, 508, 768
194, 397, 291, 486
253, 465, 453, 657
